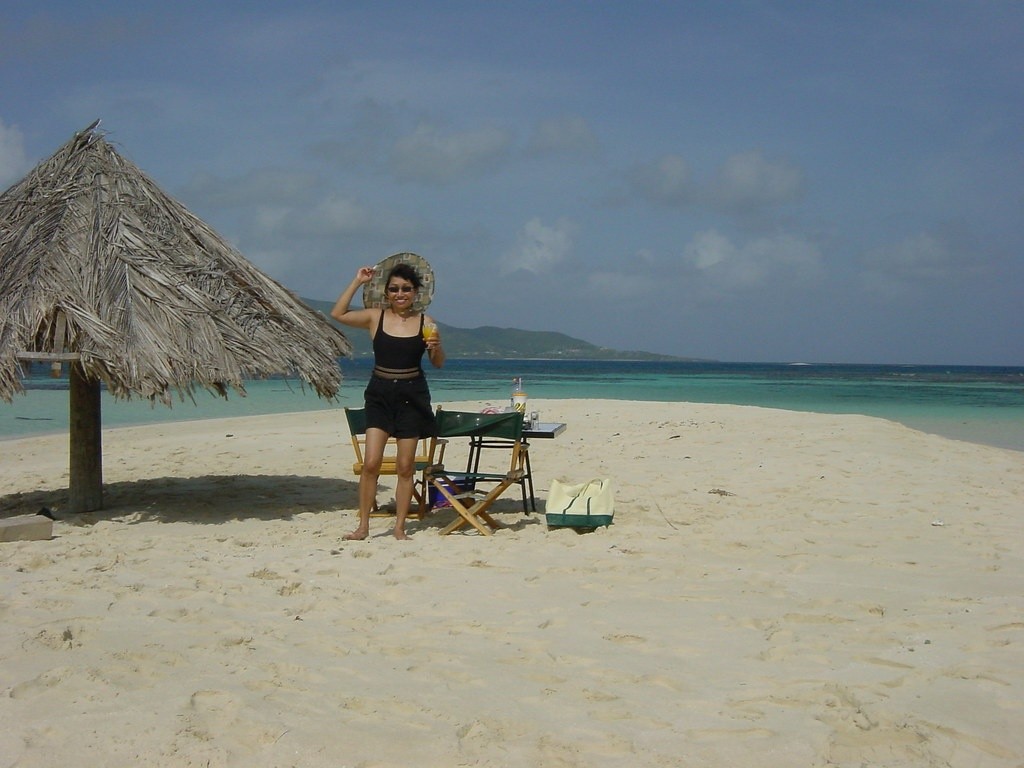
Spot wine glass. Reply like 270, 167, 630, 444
423, 325, 437, 349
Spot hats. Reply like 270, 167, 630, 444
363, 252, 435, 314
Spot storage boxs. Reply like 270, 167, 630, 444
428, 476, 475, 505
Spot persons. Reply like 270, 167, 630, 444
332, 252, 446, 540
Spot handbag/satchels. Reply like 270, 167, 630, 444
545, 478, 614, 526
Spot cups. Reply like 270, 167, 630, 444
512, 394, 528, 413
531, 412, 540, 430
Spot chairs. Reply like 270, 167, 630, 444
423, 405, 530, 536
344, 406, 429, 518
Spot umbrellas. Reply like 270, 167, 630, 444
0, 120, 353, 513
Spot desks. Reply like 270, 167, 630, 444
463, 420, 566, 517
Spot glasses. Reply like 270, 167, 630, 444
389, 288, 411, 292
